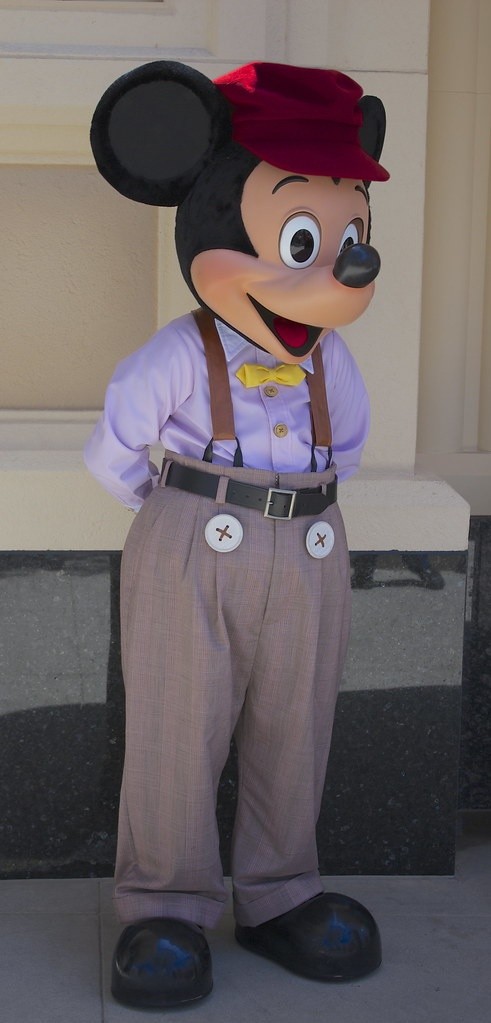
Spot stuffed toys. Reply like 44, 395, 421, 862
80, 61, 391, 1006
